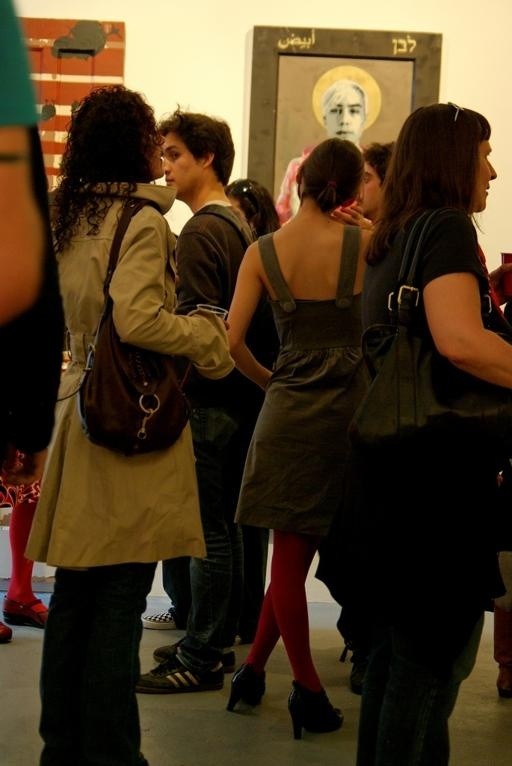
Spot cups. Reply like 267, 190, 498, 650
196, 303, 228, 324
499, 252, 512, 301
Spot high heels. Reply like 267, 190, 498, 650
226, 664, 265, 709
288, 681, 344, 739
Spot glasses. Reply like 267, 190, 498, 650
229, 178, 259, 210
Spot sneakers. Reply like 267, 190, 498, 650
136, 610, 235, 693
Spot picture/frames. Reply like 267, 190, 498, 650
246, 23, 443, 204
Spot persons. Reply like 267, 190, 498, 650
313, 101, 510, 764
273, 62, 383, 225
2, 112, 511, 741
22, 82, 239, 765
1, 0, 48, 331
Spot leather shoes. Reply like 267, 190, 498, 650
0, 593, 48, 642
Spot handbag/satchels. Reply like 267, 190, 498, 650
78, 295, 199, 455
345, 306, 512, 456
